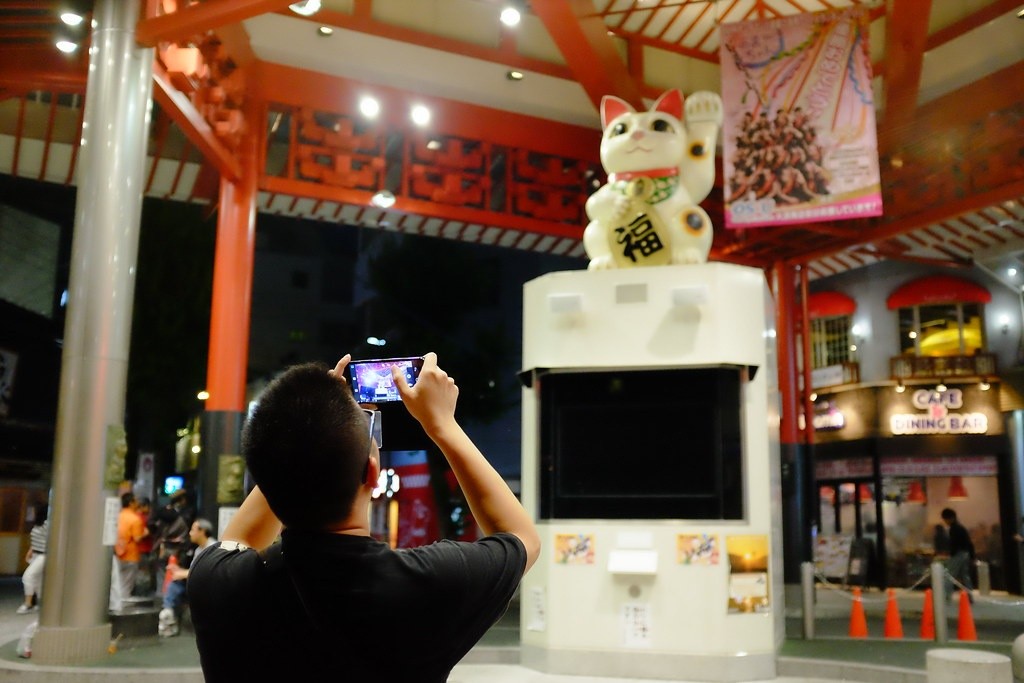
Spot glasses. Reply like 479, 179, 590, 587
362, 408, 376, 485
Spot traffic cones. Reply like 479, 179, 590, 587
959, 589, 981, 642
921, 589, 938, 640
885, 587, 908, 638
850, 585, 869, 637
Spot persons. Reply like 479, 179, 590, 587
728, 105, 829, 204
15, 485, 217, 641
860, 505, 1003, 593
187, 350, 542, 683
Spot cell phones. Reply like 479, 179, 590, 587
344, 356, 424, 403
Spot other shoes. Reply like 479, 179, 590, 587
158, 607, 179, 637
30, 605, 39, 612
16, 604, 33, 613
